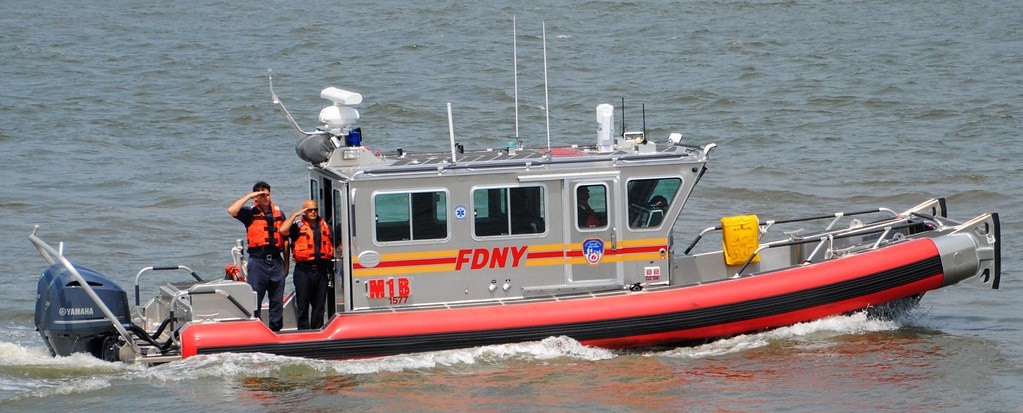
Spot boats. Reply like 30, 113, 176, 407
28, 12, 1000, 360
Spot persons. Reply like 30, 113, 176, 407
578, 186, 601, 227
227, 181, 290, 331
279, 199, 334, 330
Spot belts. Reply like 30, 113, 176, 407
248, 253, 281, 261
297, 262, 328, 270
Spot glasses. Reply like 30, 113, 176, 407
306, 208, 318, 213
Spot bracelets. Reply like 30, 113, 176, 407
290, 216, 294, 221
